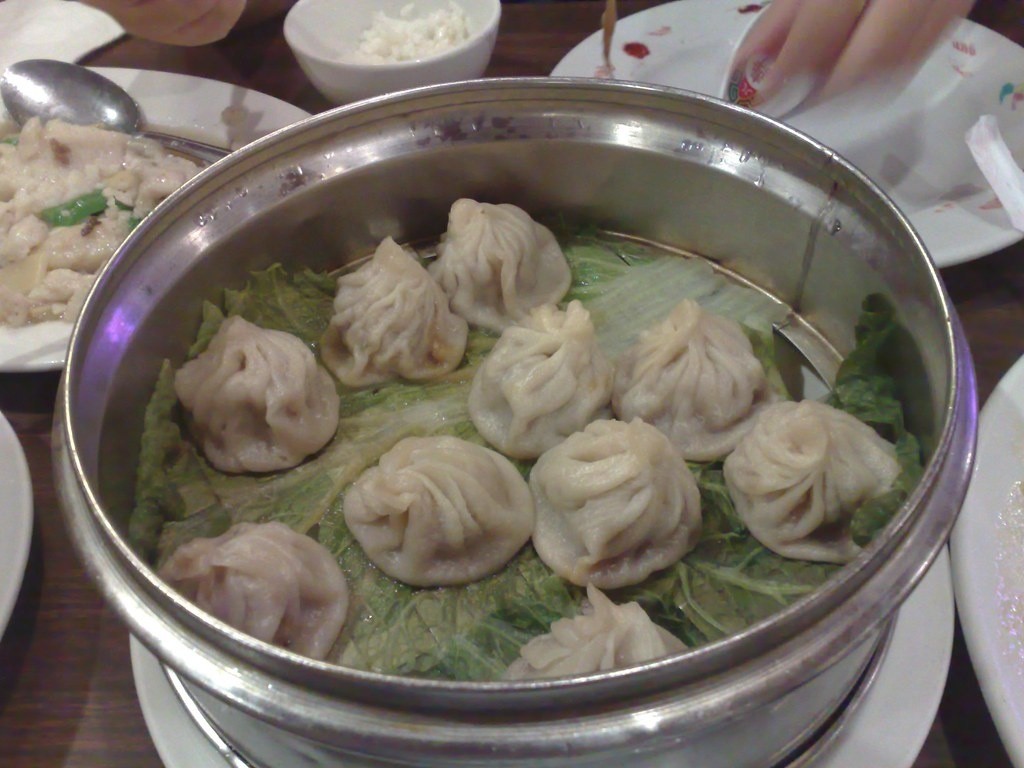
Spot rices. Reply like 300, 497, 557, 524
339, 0, 474, 64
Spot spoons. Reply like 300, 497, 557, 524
0, 58, 237, 164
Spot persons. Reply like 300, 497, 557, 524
73, 0, 973, 121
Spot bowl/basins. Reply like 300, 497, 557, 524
283, 0, 501, 107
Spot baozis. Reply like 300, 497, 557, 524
317, 237, 470, 391
607, 298, 774, 463
500, 583, 694, 680
156, 518, 349, 663
467, 298, 619, 459
525, 416, 706, 591
342, 433, 532, 586
173, 314, 340, 474
426, 196, 572, 330
721, 398, 908, 564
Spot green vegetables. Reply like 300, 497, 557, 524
35, 187, 146, 233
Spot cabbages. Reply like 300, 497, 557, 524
128, 208, 919, 682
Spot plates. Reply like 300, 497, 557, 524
948, 352, 1024, 768
130, 541, 955, 768
547, 0, 1024, 269
0, 67, 315, 373
0, 411, 35, 643
